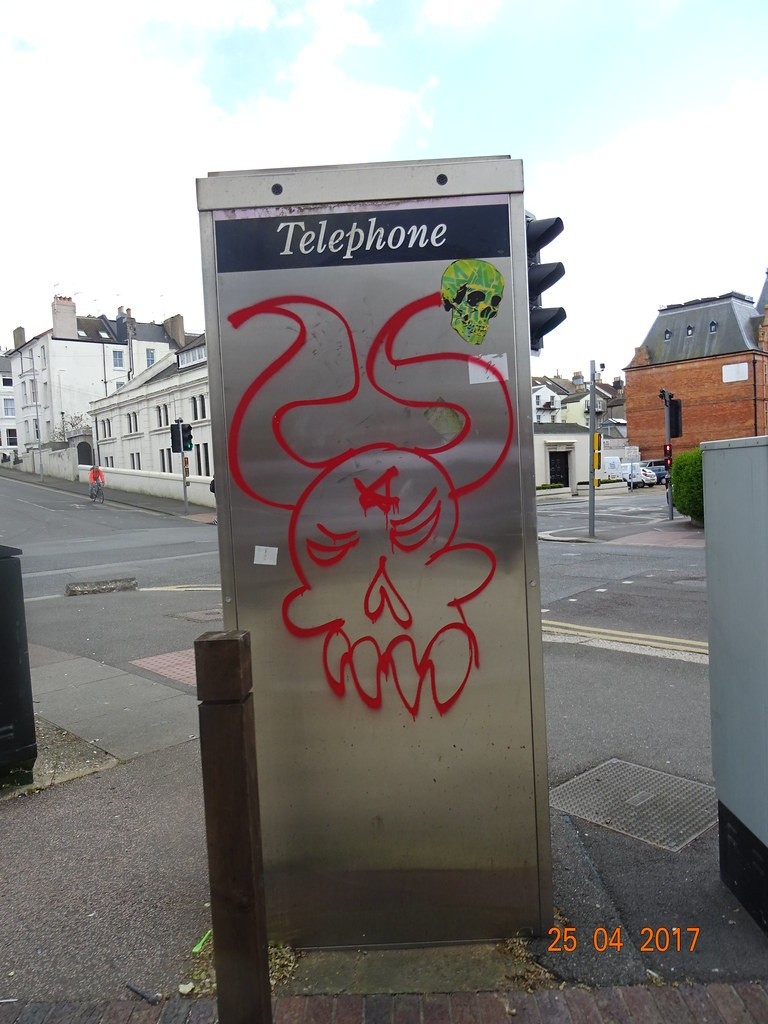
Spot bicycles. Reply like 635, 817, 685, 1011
89, 482, 105, 504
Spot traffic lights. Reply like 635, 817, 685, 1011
522, 209, 569, 357
665, 458, 672, 472
664, 444, 672, 457
181, 423, 193, 452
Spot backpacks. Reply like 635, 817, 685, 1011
90, 467, 100, 478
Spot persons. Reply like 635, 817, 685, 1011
89, 465, 104, 499
210, 475, 217, 525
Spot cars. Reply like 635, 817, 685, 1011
649, 467, 667, 485
627, 467, 657, 488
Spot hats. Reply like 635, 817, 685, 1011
95, 465, 99, 469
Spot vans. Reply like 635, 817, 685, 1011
637, 459, 671, 480
